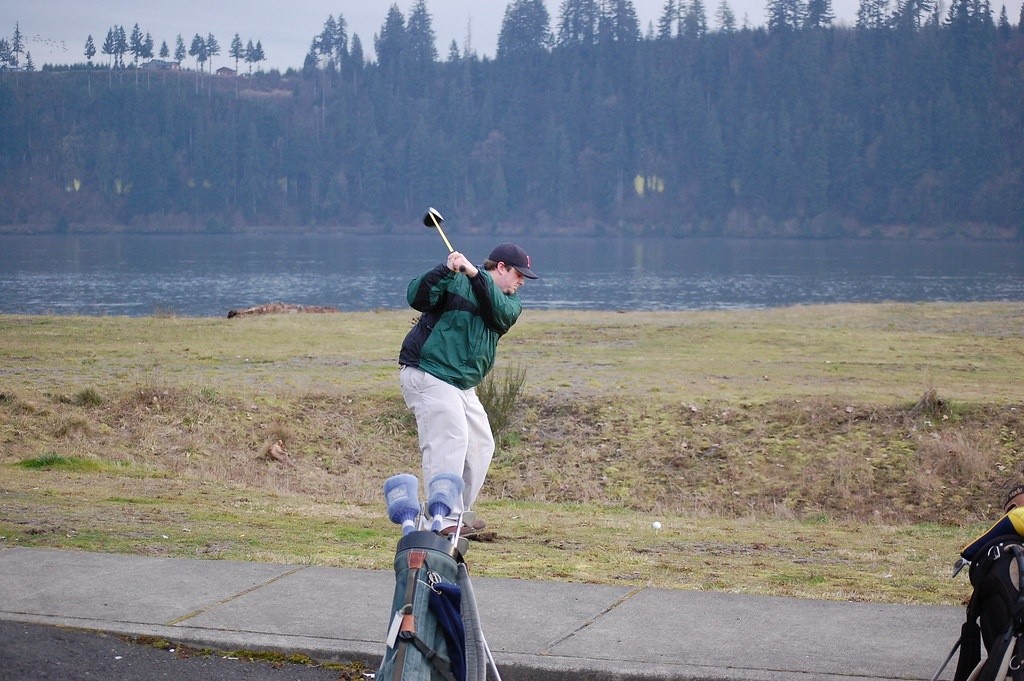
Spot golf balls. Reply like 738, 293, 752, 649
653, 521, 661, 530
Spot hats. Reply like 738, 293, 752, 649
488, 243, 538, 279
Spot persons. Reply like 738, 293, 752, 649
398, 243, 538, 542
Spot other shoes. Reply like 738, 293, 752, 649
440, 526, 477, 539
464, 519, 486, 528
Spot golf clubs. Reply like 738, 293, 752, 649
423, 207, 466, 272
418, 494, 476, 556
